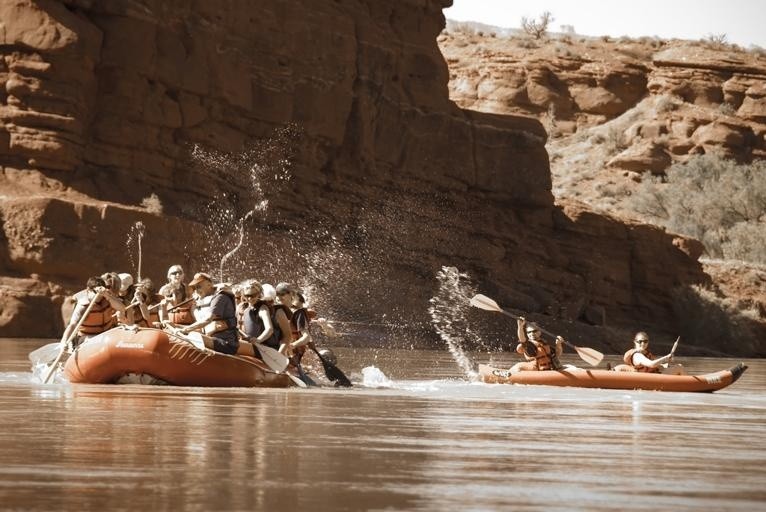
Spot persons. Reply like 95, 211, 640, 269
58, 275, 125, 356
293, 293, 336, 340
623, 331, 686, 375
117, 273, 151, 328
157, 263, 196, 327
275, 281, 310, 371
260, 283, 291, 355
516, 315, 563, 371
182, 272, 240, 355
231, 284, 242, 305
238, 280, 278, 358
100, 271, 135, 326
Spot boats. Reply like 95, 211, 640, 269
63, 325, 296, 386
478, 360, 749, 393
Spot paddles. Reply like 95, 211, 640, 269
306, 338, 353, 389
664, 335, 682, 368
468, 293, 605, 368
163, 319, 207, 355
236, 328, 291, 375
28, 338, 73, 369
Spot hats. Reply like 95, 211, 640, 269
116, 273, 133, 291
259, 283, 277, 301
188, 273, 212, 287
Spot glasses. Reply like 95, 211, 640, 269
635, 339, 649, 344
169, 271, 182, 275
244, 293, 258, 298
526, 330, 537, 334
